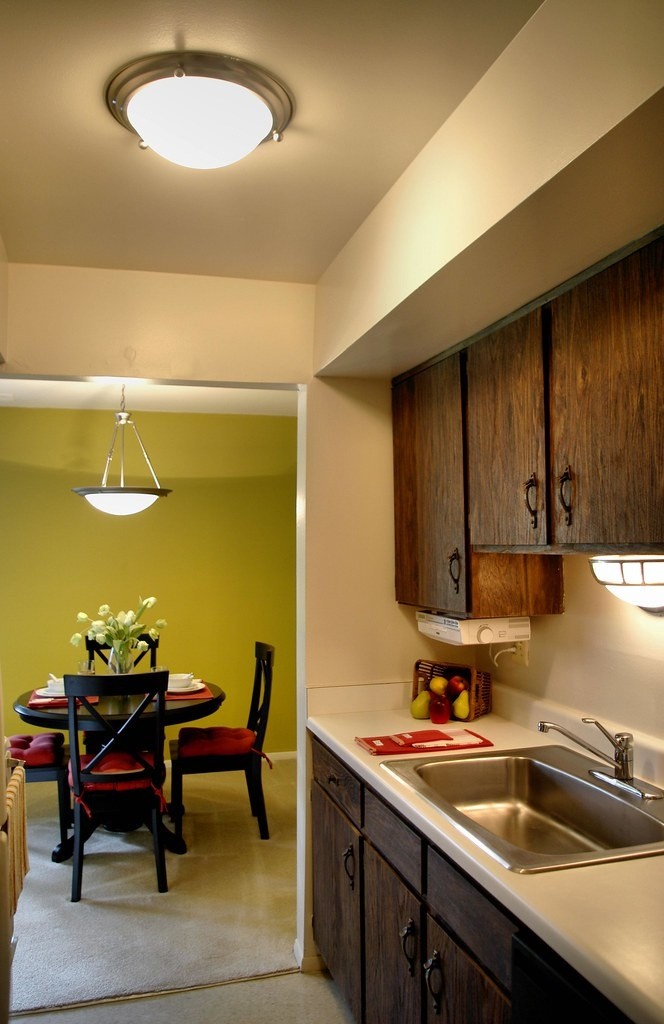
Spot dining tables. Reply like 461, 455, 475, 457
12, 674, 226, 862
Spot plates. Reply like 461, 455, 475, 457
35, 688, 66, 697
167, 683, 206, 694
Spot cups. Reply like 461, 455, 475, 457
151, 666, 167, 672
78, 660, 95, 675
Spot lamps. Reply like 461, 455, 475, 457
104, 51, 295, 171
586, 555, 664, 618
69, 382, 173, 516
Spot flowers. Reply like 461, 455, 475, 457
70, 593, 167, 656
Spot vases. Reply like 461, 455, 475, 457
111, 645, 134, 675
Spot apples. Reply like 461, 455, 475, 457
429, 677, 448, 695
448, 675, 468, 696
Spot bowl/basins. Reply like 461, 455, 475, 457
168, 674, 194, 688
47, 679, 64, 693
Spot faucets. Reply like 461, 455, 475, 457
537, 717, 634, 781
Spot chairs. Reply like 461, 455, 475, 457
62, 671, 170, 900
85, 631, 160, 674
170, 642, 274, 840
3, 732, 72, 842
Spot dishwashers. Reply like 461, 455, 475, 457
510, 929, 664, 1024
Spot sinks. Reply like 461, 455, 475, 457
380, 744, 664, 874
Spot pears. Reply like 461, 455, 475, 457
453, 688, 470, 719
410, 690, 430, 719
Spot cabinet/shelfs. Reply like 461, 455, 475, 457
309, 739, 523, 1024
391, 227, 664, 615
